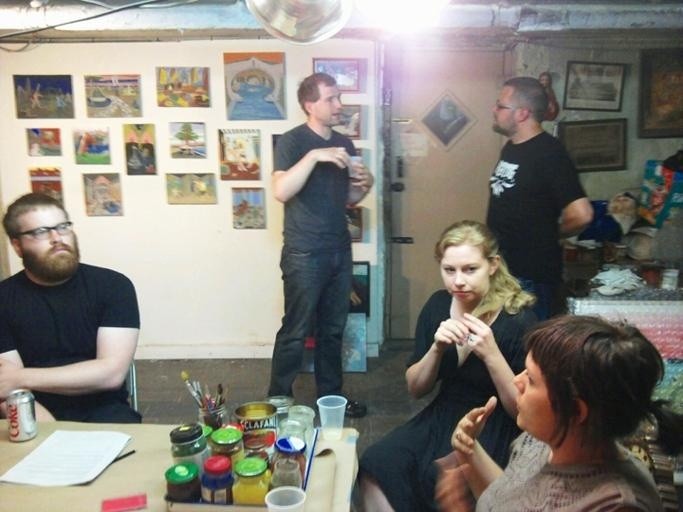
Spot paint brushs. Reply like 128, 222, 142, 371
177, 369, 227, 430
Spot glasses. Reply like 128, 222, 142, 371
18, 221, 73, 239
495, 99, 512, 111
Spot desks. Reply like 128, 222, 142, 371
0, 419, 358, 512
558, 287, 683, 473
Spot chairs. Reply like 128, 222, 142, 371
622, 408, 679, 512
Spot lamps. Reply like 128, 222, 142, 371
245, 0, 350, 46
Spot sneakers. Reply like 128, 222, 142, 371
315, 394, 367, 419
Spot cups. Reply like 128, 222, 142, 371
317, 395, 348, 441
264, 486, 307, 512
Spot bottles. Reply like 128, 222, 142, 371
278, 418, 308, 461
6, 389, 37, 443
201, 425, 211, 459
288, 405, 315, 448
245, 438, 270, 470
201, 456, 235, 505
165, 461, 201, 504
170, 423, 210, 482
232, 458, 271, 507
270, 458, 302, 490
210, 428, 244, 478
271, 436, 307, 487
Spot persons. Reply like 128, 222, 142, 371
540, 71, 560, 121
141, 132, 155, 173
356, 220, 540, 512
126, 132, 141, 173
0, 193, 141, 425
451, 320, 664, 511
29, 91, 44, 109
91, 177, 120, 216
484, 76, 594, 315
268, 74, 373, 419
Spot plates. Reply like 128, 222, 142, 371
164, 426, 318, 512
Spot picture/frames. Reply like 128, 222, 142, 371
331, 105, 362, 140
417, 88, 479, 153
313, 58, 358, 94
348, 262, 370, 318
298, 312, 367, 373
562, 60, 630, 111
636, 46, 682, 139
558, 118, 628, 174
344, 208, 363, 241
